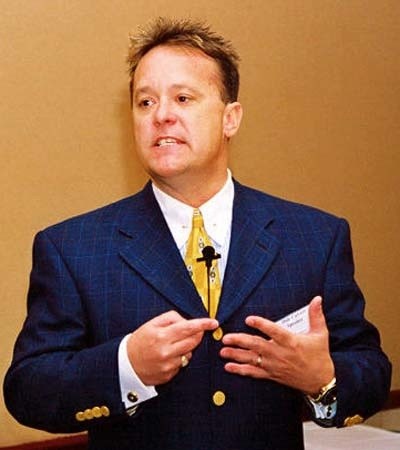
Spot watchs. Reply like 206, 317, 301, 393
307, 377, 337, 407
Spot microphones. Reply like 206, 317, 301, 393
196, 246, 222, 267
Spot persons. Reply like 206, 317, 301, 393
3, 18, 392, 450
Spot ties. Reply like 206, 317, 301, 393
184, 208, 221, 318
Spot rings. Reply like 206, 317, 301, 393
256, 355, 262, 367
181, 356, 189, 367
